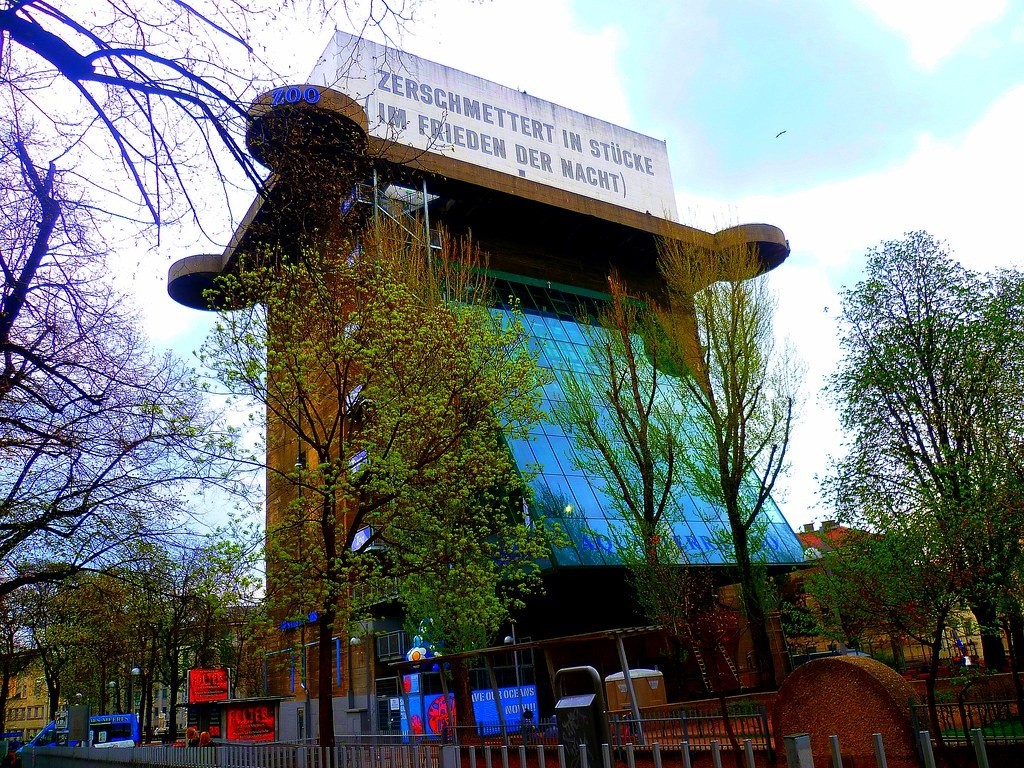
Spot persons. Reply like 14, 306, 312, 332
6, 735, 35, 750
187, 727, 215, 746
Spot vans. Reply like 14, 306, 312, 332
15, 713, 142, 756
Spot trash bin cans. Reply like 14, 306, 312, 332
555, 692, 603, 768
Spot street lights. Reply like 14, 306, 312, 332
127, 656, 141, 714
501, 623, 528, 750
350, 619, 372, 735
